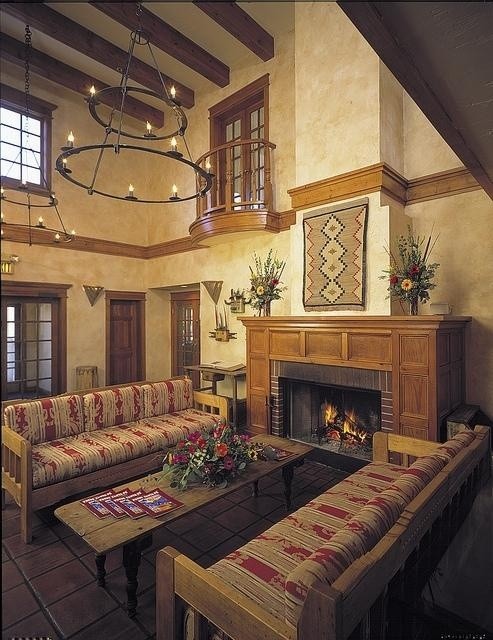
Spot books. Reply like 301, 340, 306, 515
128, 487, 185, 519
98, 487, 133, 519
110, 486, 148, 520
79, 489, 116, 520
274, 449, 296, 462
198, 359, 245, 371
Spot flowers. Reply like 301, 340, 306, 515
245, 248, 288, 310
377, 221, 440, 304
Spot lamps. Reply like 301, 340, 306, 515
0, 24, 73, 246
55, 0, 215, 203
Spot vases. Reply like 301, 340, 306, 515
266, 301, 270, 316
410, 296, 418, 316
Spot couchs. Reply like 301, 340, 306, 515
155, 425, 493, 639
0, 375, 229, 544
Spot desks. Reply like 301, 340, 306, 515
183, 365, 246, 432
